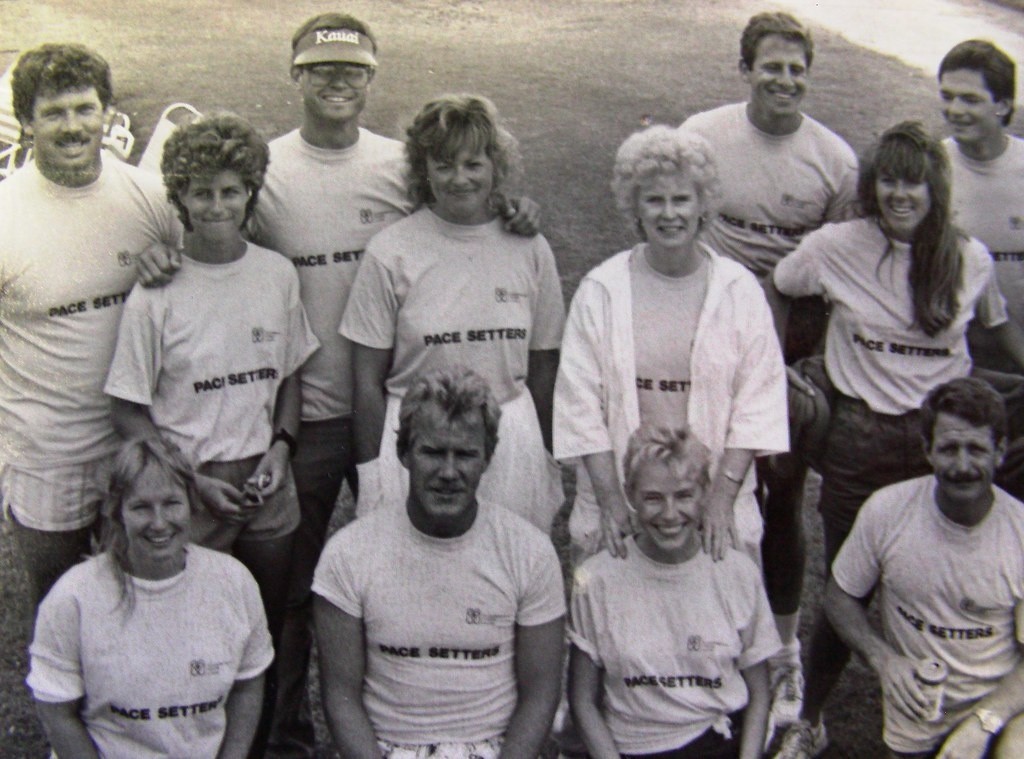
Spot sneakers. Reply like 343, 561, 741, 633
773, 711, 829, 759
768, 664, 804, 726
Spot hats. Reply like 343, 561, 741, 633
291, 27, 378, 68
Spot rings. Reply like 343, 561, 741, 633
535, 216, 542, 221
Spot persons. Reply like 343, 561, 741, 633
0, 44, 319, 759
762, 41, 1024, 759
241, 12, 568, 759
551, 12, 868, 759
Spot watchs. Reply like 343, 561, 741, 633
973, 708, 1005, 736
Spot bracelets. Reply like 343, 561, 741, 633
718, 459, 745, 490
270, 428, 297, 459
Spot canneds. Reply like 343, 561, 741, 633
914, 657, 947, 720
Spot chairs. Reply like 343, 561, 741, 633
0, 63, 204, 181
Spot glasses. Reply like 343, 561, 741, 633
299, 62, 372, 90
